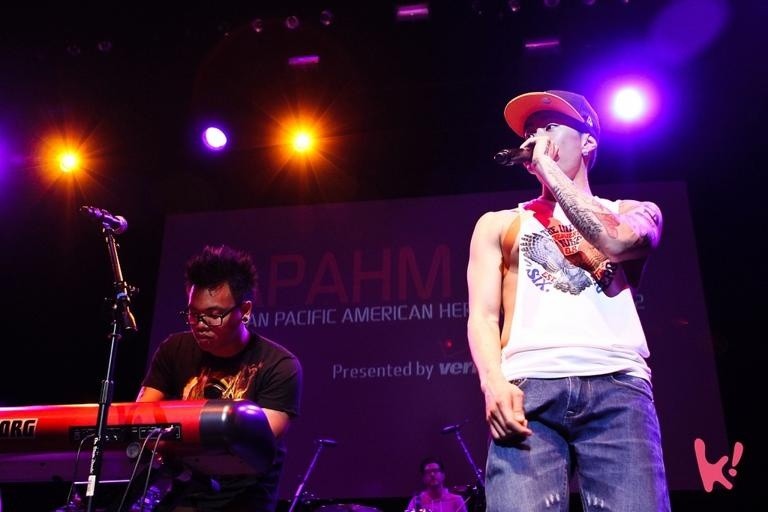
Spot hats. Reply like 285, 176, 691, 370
503, 89, 601, 145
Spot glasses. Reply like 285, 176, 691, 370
179, 298, 246, 327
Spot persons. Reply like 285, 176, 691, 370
464, 87, 670, 512
404, 457, 468, 512
105, 241, 303, 512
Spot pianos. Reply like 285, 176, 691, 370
0, 400, 289, 483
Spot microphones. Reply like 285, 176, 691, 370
316, 439, 338, 448
494, 146, 533, 166
440, 422, 461, 435
82, 206, 128, 236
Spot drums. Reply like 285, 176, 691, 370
318, 504, 379, 512
